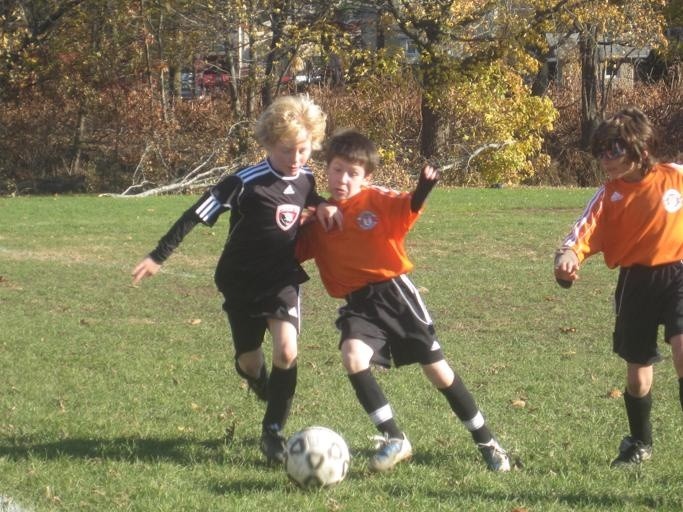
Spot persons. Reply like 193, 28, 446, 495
293, 132, 510, 472
130, 93, 345, 465
554, 107, 683, 469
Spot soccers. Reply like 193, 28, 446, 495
283, 426, 349, 491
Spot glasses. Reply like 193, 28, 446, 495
591, 137, 627, 161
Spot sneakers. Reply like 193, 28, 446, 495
611, 434, 652, 470
476, 438, 510, 473
259, 426, 286, 464
248, 370, 271, 401
366, 430, 413, 473
